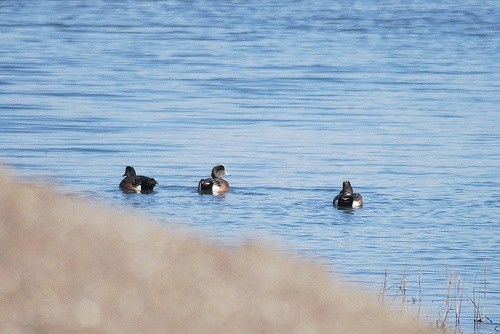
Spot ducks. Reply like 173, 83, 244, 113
332, 180, 363, 210
197, 164, 232, 196
119, 166, 159, 195
